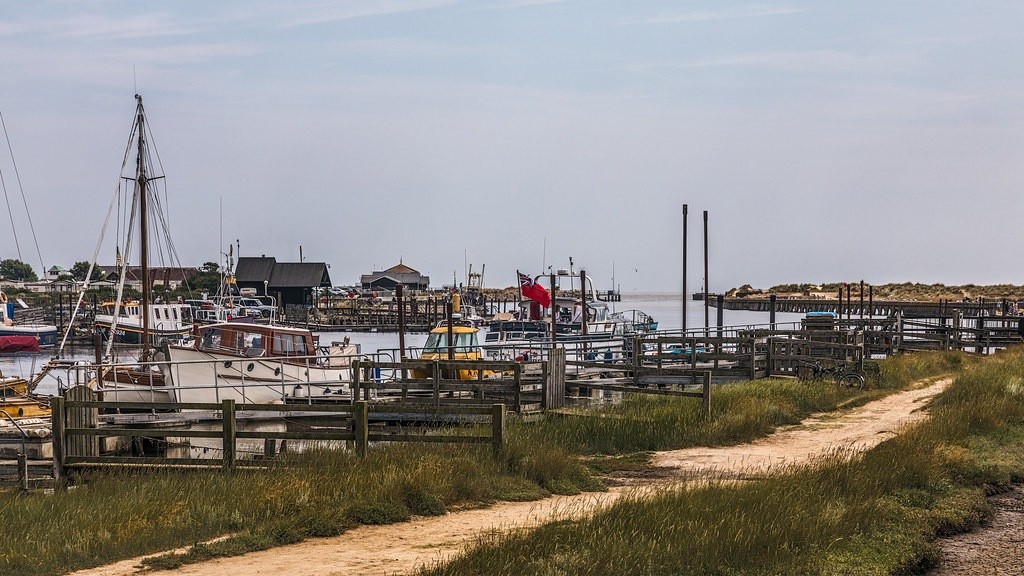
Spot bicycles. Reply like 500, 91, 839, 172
815, 358, 865, 392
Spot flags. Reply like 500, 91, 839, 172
519, 273, 550, 309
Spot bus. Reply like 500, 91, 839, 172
54, 304, 84, 317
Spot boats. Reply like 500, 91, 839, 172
410, 264, 496, 379
483, 320, 550, 361
154, 323, 362, 420
95, 296, 194, 344
0, 291, 58, 348
485, 257, 658, 342
0, 376, 52, 419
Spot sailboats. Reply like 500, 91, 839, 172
48, 93, 189, 419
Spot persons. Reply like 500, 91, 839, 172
962, 297, 1018, 317
646, 316, 655, 324
555, 305, 563, 322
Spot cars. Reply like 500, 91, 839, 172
312, 283, 353, 298
185, 294, 278, 321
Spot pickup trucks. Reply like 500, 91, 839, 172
644, 344, 704, 361
